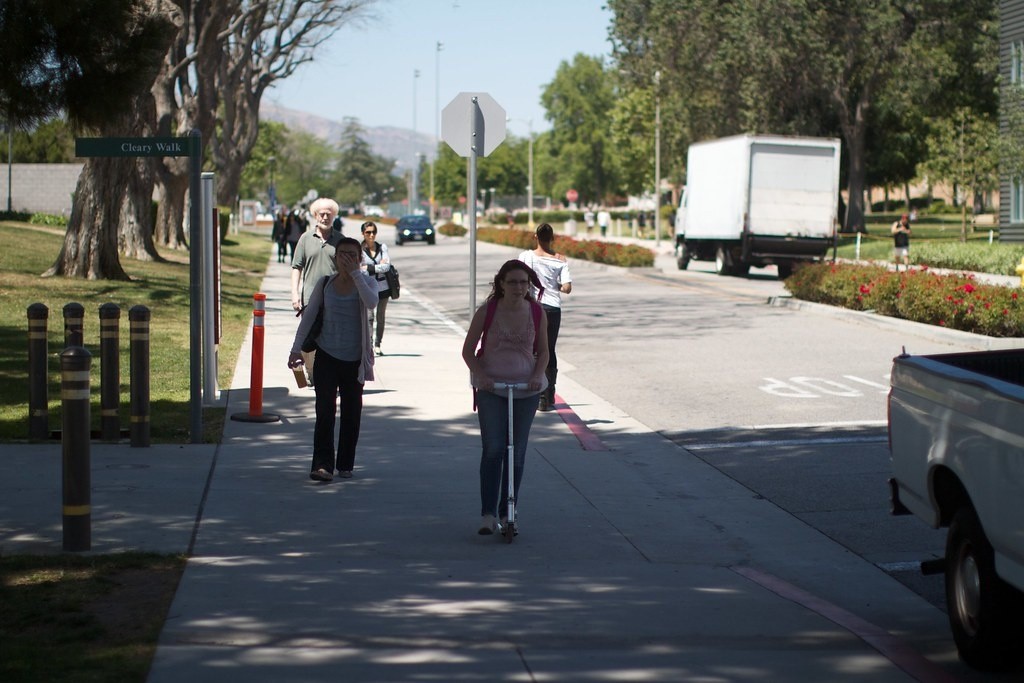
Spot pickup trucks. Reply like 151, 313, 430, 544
886, 345, 1023, 676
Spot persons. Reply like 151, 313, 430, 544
462, 260, 549, 537
271, 203, 309, 264
564, 213, 578, 237
518, 223, 571, 411
597, 205, 610, 238
891, 212, 912, 271
291, 197, 346, 387
584, 207, 594, 235
360, 221, 390, 355
288, 240, 379, 481
332, 216, 343, 231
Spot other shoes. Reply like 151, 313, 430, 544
478, 517, 497, 533
339, 470, 352, 478
309, 468, 333, 481
500, 517, 519, 534
375, 347, 384, 356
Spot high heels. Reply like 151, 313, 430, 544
539, 396, 548, 411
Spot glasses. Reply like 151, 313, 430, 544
501, 280, 529, 287
364, 231, 376, 234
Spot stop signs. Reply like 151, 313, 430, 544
566, 189, 578, 202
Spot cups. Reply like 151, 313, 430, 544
290, 360, 307, 388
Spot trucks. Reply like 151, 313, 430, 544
671, 132, 842, 277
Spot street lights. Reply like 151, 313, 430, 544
410, 68, 423, 209
618, 68, 661, 248
505, 115, 534, 225
431, 41, 445, 218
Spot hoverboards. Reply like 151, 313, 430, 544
486, 383, 534, 545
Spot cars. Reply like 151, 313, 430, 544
395, 215, 436, 245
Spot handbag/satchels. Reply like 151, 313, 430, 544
385, 264, 400, 300
